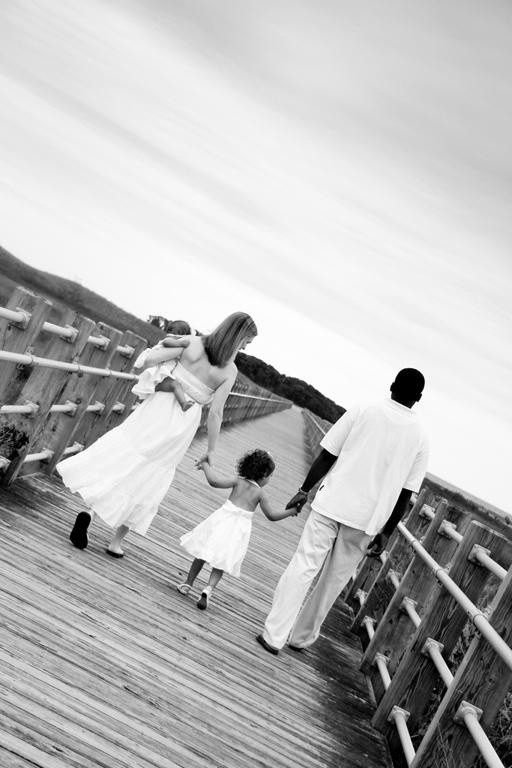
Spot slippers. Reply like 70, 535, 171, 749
287, 642, 304, 651
256, 633, 279, 655
70, 511, 92, 550
106, 546, 125, 558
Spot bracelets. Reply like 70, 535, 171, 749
298, 487, 308, 496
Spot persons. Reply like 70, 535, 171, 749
255, 364, 430, 657
130, 315, 196, 413
176, 446, 300, 610
53, 310, 258, 558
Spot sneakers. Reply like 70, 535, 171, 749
287, 643, 288, 644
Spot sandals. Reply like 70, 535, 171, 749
178, 584, 192, 594
197, 587, 213, 610
